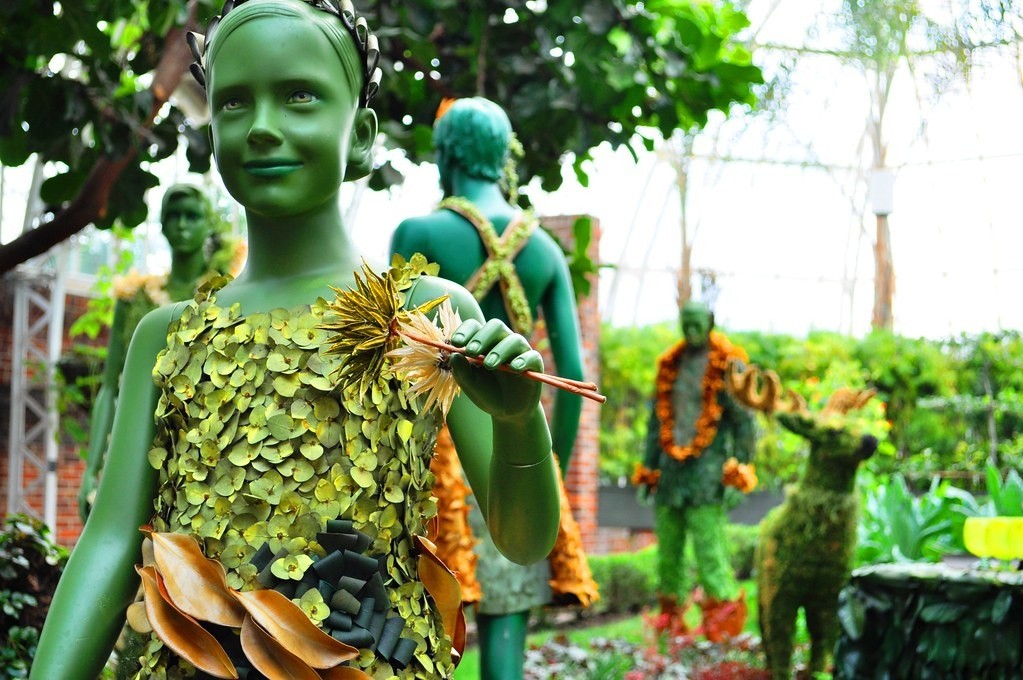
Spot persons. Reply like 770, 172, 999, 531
29, 0, 560, 680
390, 95, 586, 680
77, 184, 220, 523
636, 303, 756, 645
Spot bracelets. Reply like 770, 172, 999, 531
632, 465, 660, 492
724, 457, 757, 493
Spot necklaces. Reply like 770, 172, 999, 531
657, 334, 725, 459
